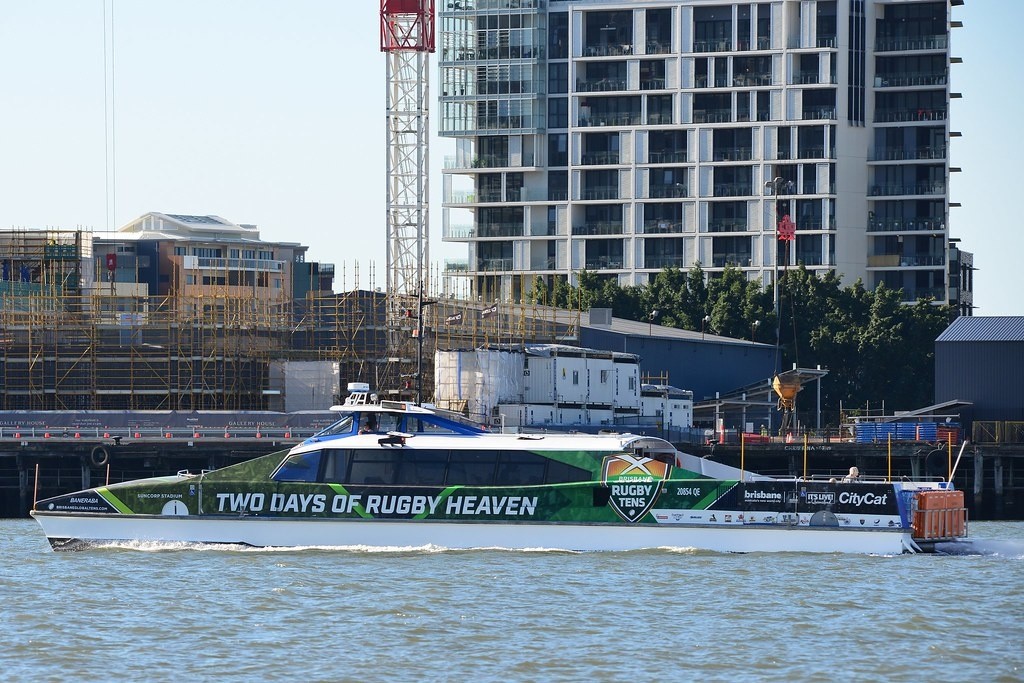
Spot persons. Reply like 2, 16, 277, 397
364, 422, 373, 432
760, 425, 767, 436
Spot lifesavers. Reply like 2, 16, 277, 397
91, 445, 110, 467
925, 449, 952, 476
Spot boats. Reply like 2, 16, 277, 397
26, 382, 972, 556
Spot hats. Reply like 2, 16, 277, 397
760, 424, 764, 428
850, 467, 858, 474
